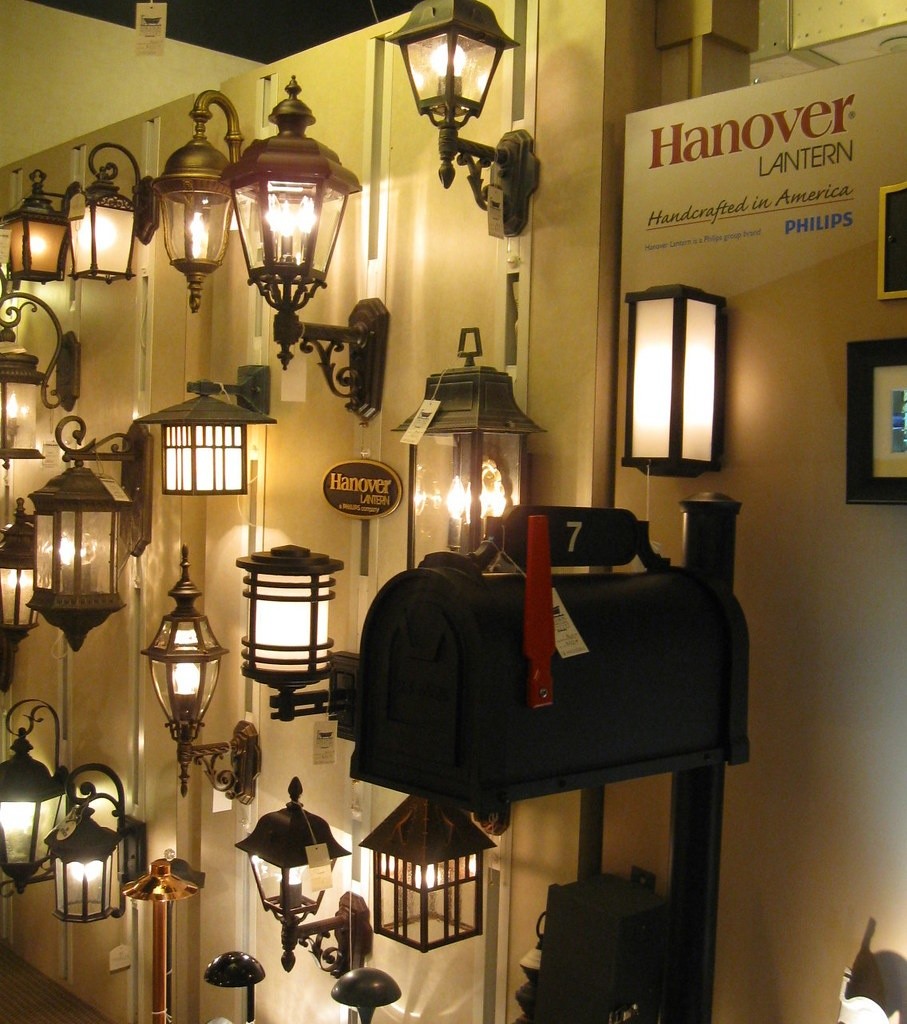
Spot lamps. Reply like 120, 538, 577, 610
838, 949, 891, 1024
370, 0, 541, 239
0, 74, 727, 1024
